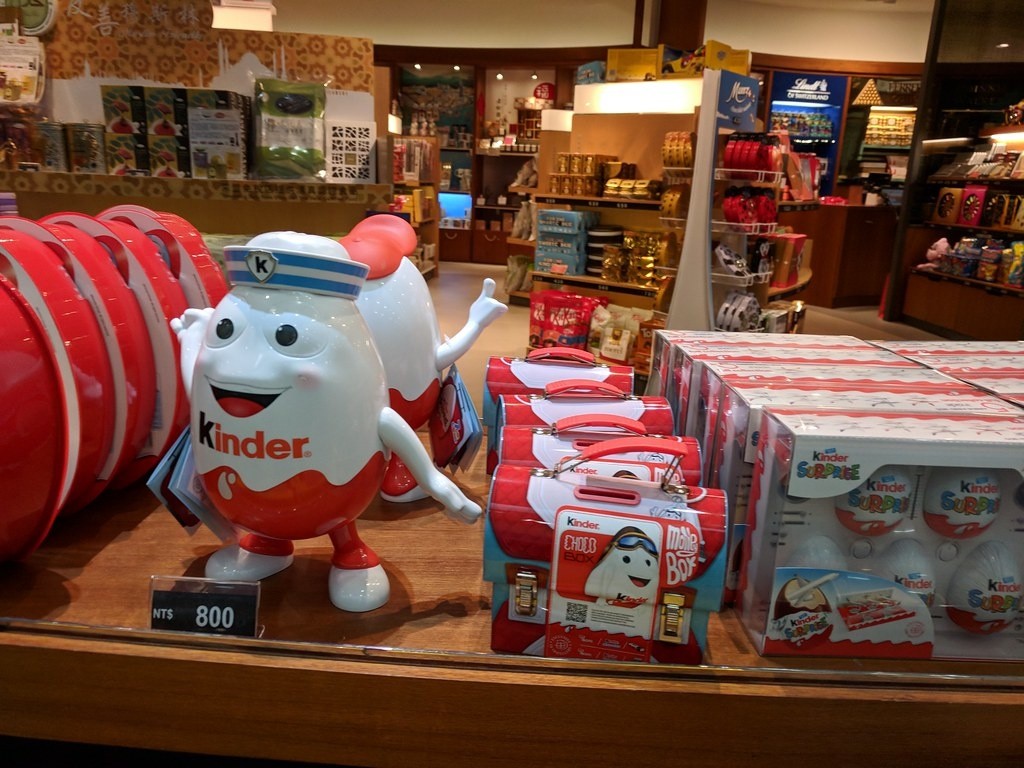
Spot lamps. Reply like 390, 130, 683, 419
209, 0, 278, 32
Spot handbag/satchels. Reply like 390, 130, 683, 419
496, 412, 704, 489
495, 379, 674, 436
481, 439, 729, 666
481, 346, 633, 474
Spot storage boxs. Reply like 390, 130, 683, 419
188, 89, 251, 179
390, 153, 664, 275
906, 354, 1024, 373
575, 61, 607, 84
952, 372, 1024, 394
709, 382, 1024, 613
100, 85, 144, 133
648, 328, 884, 398
37, 122, 64, 172
740, 407, 1024, 662
536, 209, 602, 235
767, 228, 808, 288
144, 85, 185, 135
939, 253, 980, 278
65, 122, 104, 174
105, 131, 146, 177
535, 232, 588, 253
685, 359, 977, 489
1000, 393, 1024, 409
864, 339, 1024, 355
668, 342, 926, 444
147, 135, 188, 178
532, 252, 592, 274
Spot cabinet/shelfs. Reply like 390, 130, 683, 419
472, 57, 563, 267
884, 124, 1024, 341
392, 134, 443, 277
528, 38, 817, 395
